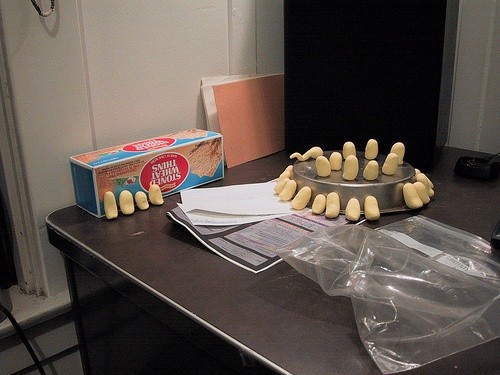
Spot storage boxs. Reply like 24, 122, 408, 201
68, 126, 225, 218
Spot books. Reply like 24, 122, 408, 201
200, 71, 285, 169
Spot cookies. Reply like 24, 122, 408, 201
185, 137, 222, 178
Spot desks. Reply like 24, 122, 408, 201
45, 147, 500, 375
